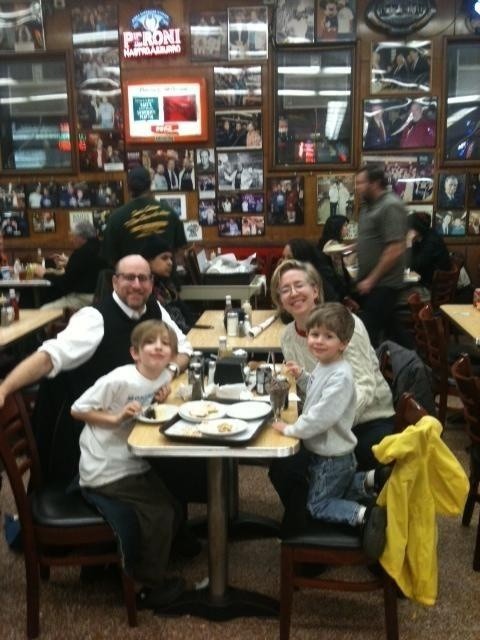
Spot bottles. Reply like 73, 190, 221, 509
0, 288, 19, 327
13, 258, 32, 281
224, 294, 251, 336
187, 351, 205, 400
217, 336, 228, 359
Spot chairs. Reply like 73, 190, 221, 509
1, 392, 140, 637
277, 392, 436, 639
407, 286, 480, 575
1, 245, 479, 450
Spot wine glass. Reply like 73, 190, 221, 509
271, 377, 288, 424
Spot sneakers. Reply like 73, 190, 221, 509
374, 463, 394, 495
359, 503, 386, 559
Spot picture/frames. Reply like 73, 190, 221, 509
0, 0, 480, 238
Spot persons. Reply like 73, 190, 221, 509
271, 301, 390, 531
108, 165, 186, 258
278, 166, 451, 339
269, 258, 398, 513
0, 182, 91, 235
147, 163, 264, 234
32, 222, 105, 298
1, 254, 193, 480
70, 318, 188, 611
139, 234, 192, 331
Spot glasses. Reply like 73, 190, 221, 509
118, 272, 148, 282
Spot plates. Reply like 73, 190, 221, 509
134, 400, 272, 437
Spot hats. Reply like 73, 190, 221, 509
139, 236, 172, 259
127, 166, 150, 190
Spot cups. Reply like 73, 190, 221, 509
1, 269, 11, 280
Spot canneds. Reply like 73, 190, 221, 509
257, 365, 271, 395
188, 362, 203, 384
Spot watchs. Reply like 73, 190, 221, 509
168, 364, 178, 377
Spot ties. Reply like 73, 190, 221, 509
171, 171, 178, 186
